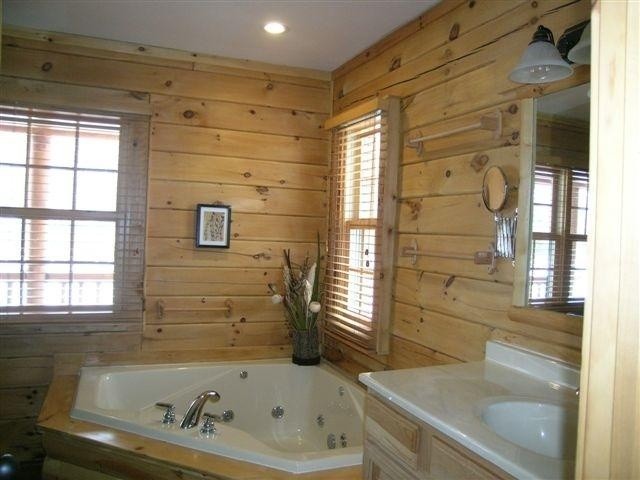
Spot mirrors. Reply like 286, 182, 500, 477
518, 82, 589, 317
481, 166, 508, 214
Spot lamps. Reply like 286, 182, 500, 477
505, 15, 591, 88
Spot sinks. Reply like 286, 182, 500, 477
482, 401, 578, 459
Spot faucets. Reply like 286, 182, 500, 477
178, 389, 221, 430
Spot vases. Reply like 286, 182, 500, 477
291, 327, 320, 366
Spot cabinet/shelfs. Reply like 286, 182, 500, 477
358, 386, 518, 479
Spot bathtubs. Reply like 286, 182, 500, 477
69, 357, 365, 475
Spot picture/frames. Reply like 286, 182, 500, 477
195, 203, 231, 249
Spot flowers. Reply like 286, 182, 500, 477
267, 230, 325, 328
